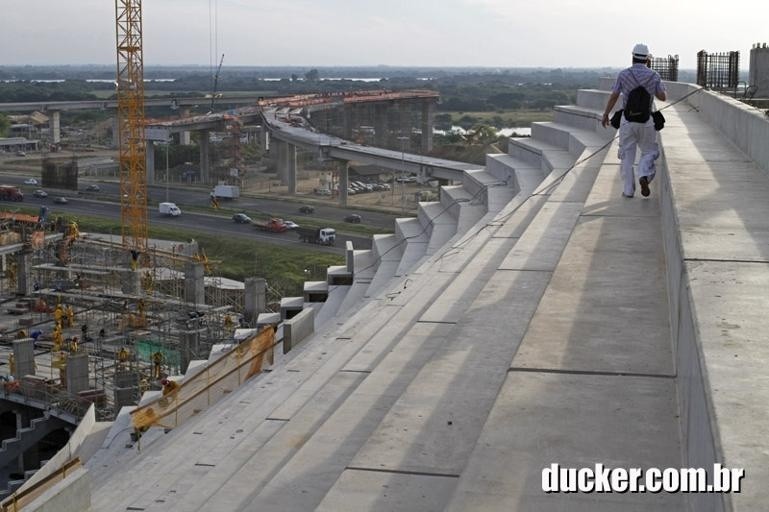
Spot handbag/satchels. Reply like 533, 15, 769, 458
652, 110, 666, 131
609, 108, 623, 129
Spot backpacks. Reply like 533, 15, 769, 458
623, 85, 654, 124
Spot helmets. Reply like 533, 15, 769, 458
632, 43, 650, 60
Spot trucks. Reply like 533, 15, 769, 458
158, 201, 181, 217
0, 185, 23, 202
294, 223, 337, 246
210, 185, 239, 200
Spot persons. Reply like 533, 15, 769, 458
7, 294, 183, 405
223, 312, 233, 332
191, 251, 211, 273
600, 42, 667, 200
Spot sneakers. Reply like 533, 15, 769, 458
638, 175, 651, 197
622, 191, 633, 198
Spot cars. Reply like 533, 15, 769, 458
14, 150, 25, 157
53, 196, 67, 204
227, 181, 392, 234
85, 184, 99, 192
31, 189, 47, 198
22, 178, 38, 186
